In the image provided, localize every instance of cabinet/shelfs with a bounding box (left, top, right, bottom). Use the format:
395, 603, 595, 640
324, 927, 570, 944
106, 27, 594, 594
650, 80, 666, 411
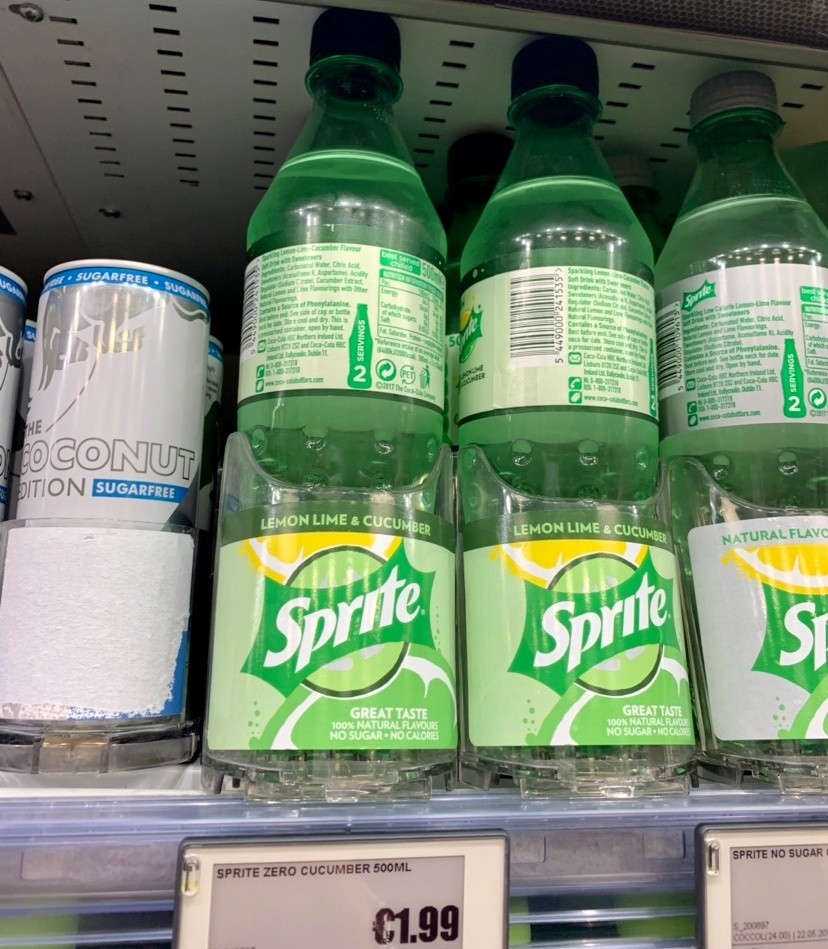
0, 0, 828, 949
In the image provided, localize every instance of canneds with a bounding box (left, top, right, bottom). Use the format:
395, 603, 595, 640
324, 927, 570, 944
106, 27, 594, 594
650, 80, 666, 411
0, 261, 225, 734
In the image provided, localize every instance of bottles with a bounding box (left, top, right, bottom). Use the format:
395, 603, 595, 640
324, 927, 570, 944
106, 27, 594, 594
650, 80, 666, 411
622, 185, 670, 265
237, 7, 450, 779
658, 68, 828, 753
447, 128, 514, 521
460, 34, 698, 782
